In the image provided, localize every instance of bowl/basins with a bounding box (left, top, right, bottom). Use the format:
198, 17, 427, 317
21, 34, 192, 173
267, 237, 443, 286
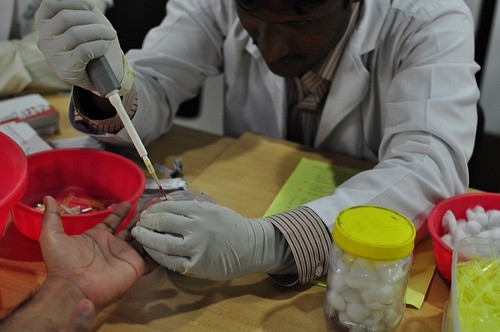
427, 193, 500, 281
13, 147, 146, 242
0, 131, 29, 238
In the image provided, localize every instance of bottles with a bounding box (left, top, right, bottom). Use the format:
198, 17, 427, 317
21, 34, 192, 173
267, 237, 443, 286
324, 206, 414, 332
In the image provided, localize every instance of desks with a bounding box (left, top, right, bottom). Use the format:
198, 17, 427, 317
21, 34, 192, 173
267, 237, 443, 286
1, 93, 480, 332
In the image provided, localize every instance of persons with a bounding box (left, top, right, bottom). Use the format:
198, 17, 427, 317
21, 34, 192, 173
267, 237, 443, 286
0, 196, 161, 332
34, 0, 480, 287
0, 0, 114, 96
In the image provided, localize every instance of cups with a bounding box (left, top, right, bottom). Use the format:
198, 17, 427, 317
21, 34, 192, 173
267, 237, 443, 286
450, 238, 500, 332
